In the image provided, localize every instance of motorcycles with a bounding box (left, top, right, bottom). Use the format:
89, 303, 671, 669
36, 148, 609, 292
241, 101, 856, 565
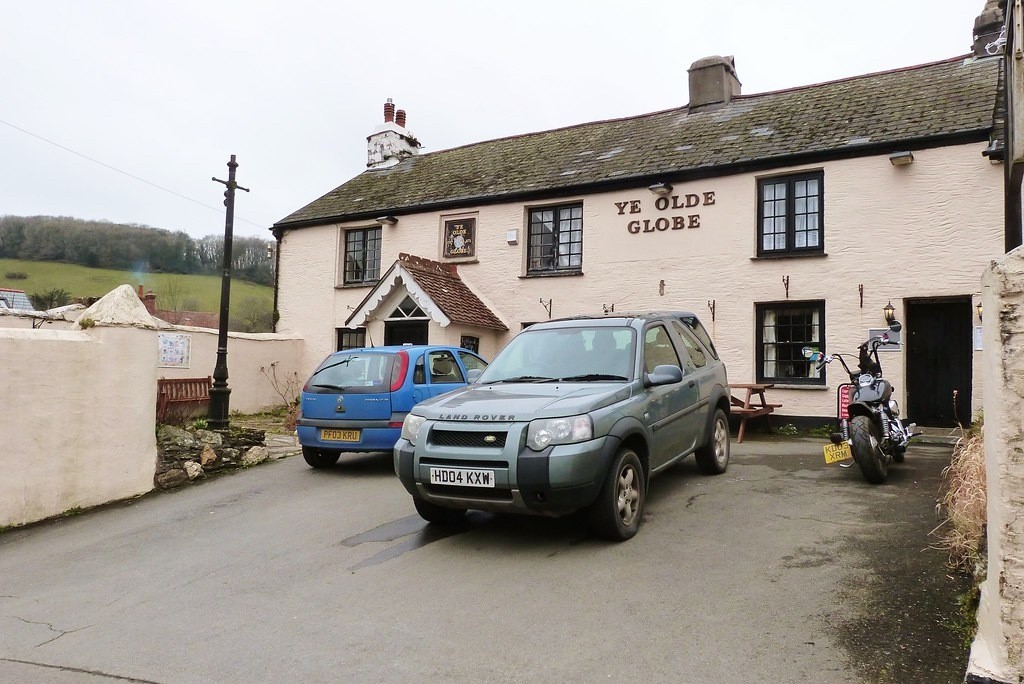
802, 321, 922, 484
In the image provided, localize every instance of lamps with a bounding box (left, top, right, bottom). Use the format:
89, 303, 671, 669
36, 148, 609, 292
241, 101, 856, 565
975, 302, 982, 322
889, 150, 914, 165
882, 301, 895, 326
649, 181, 674, 195
375, 216, 398, 225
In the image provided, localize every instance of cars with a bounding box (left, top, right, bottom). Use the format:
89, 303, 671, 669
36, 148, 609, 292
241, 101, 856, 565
297, 343, 488, 469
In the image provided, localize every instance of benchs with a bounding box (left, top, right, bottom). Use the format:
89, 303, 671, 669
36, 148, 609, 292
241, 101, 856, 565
729, 383, 774, 443
588, 328, 690, 375
157, 375, 212, 422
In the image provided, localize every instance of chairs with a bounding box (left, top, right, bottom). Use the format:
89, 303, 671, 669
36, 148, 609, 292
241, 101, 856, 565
432, 359, 457, 382
620, 341, 654, 377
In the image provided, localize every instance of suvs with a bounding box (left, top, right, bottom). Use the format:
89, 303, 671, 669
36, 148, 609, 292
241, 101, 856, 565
393, 310, 731, 542
